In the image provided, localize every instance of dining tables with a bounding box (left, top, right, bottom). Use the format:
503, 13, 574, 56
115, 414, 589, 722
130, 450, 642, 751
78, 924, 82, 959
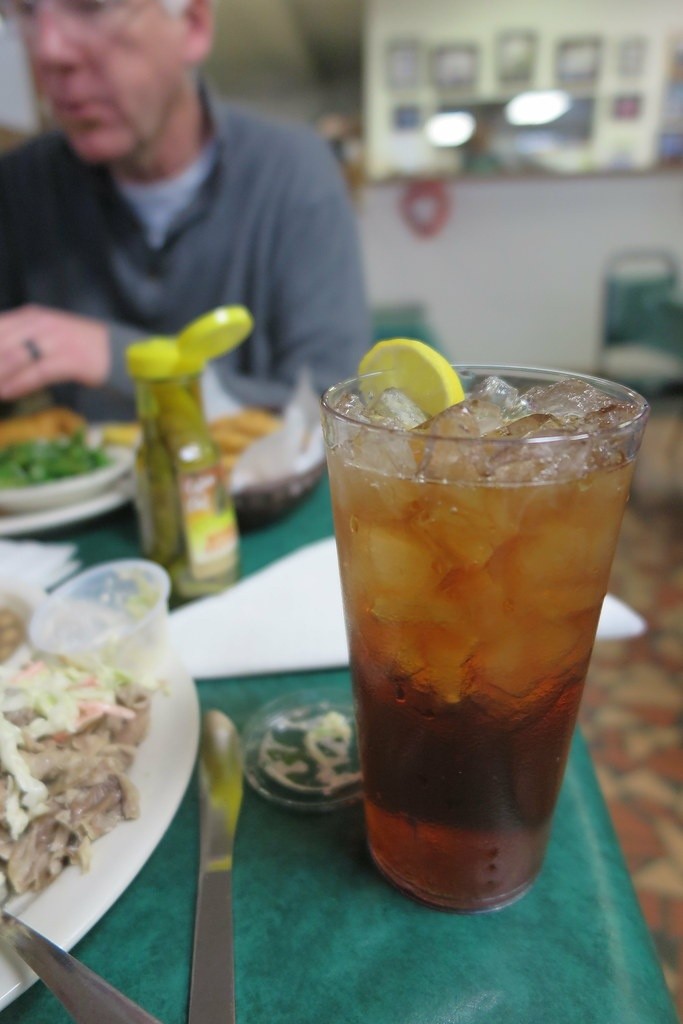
0, 316, 681, 1024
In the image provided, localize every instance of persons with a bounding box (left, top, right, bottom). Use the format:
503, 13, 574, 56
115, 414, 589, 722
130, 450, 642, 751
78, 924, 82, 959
0, 1, 372, 429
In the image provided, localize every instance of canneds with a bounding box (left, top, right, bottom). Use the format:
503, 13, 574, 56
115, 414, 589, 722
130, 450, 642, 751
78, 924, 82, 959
123, 309, 261, 597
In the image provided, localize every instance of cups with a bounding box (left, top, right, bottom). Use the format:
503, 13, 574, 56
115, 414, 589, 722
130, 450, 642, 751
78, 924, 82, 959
319, 363, 654, 912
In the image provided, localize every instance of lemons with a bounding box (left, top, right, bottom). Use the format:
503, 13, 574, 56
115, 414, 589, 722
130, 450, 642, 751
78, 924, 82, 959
358, 335, 463, 412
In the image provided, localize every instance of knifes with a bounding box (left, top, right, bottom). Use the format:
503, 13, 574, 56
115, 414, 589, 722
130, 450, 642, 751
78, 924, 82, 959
185, 706, 247, 1023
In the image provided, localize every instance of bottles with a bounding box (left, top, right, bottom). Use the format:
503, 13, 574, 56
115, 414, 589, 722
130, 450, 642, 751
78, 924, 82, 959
124, 333, 240, 602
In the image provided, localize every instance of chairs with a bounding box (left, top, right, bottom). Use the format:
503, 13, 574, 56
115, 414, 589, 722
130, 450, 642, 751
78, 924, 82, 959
588, 245, 681, 498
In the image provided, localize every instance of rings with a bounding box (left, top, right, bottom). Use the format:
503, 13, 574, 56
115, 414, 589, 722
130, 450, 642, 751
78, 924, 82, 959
24, 336, 43, 363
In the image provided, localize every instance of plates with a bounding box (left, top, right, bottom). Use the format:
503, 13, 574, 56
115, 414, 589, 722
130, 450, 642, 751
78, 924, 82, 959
0, 445, 133, 515
0, 472, 136, 543
0, 579, 202, 1014
